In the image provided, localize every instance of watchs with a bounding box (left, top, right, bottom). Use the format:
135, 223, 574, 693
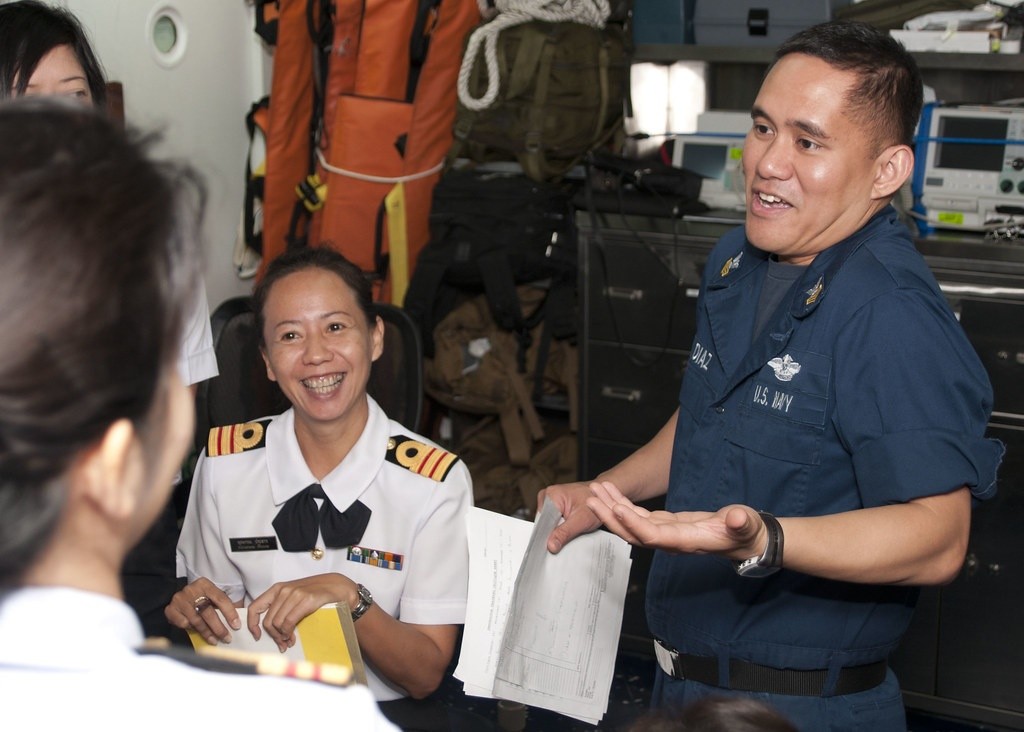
351, 584, 373, 622
730, 510, 784, 578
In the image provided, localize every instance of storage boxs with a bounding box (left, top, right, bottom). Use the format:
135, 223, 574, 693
692, 1, 854, 48
632, 0, 697, 44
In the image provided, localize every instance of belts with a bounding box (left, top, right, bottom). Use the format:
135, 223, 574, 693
650, 639, 893, 700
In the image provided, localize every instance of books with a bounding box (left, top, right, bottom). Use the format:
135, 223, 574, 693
185, 601, 370, 685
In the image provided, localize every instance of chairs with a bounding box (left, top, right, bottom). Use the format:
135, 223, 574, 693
119, 294, 423, 653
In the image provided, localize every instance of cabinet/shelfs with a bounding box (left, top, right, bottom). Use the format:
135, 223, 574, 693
885, 230, 1023, 728
576, 207, 747, 730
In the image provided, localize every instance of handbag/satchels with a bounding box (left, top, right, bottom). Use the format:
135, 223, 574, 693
454, 15, 633, 169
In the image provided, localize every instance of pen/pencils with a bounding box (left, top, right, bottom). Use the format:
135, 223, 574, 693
194, 585, 235, 614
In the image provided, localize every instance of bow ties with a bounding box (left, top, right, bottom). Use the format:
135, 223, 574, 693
272, 484, 372, 552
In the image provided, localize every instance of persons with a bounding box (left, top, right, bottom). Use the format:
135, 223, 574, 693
537, 19, 1006, 732
165, 245, 487, 731
1, 0, 220, 649
0, 94, 406, 730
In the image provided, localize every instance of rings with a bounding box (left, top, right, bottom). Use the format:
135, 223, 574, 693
192, 596, 209, 607
195, 600, 212, 614
